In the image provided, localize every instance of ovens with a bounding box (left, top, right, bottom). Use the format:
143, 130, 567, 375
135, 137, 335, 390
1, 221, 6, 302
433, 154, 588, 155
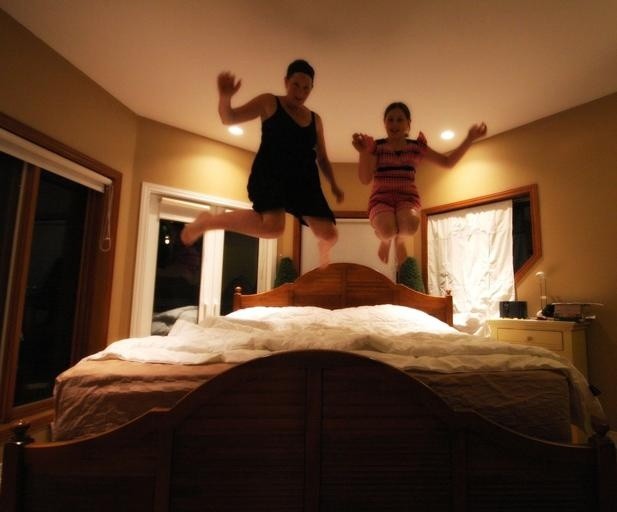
0, 262, 616, 512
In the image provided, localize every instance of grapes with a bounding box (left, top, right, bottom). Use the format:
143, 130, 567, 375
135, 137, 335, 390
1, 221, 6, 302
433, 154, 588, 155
482, 317, 589, 385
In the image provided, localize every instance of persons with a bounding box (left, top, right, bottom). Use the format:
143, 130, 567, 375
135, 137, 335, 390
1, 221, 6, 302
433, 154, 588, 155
180, 57, 344, 269
349, 100, 488, 270
